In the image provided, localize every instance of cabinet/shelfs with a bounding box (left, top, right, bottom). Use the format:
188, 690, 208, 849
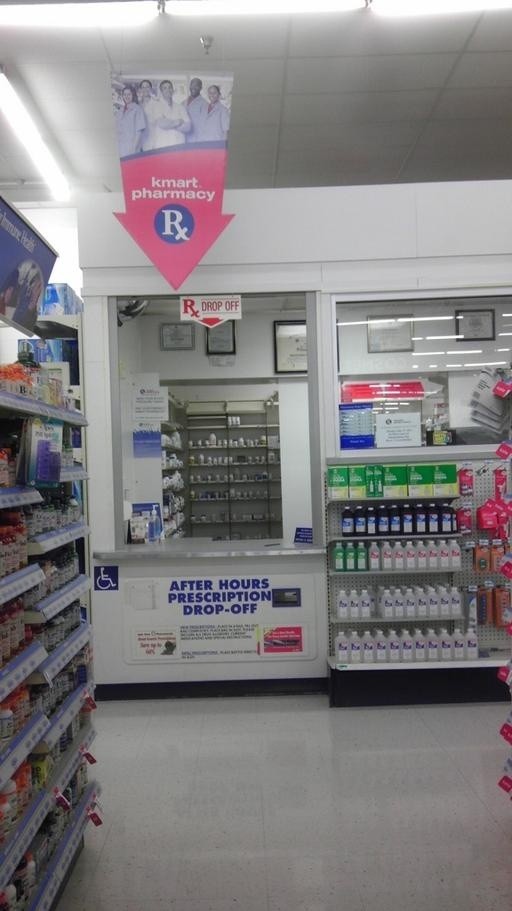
0, 191, 102, 911
134, 394, 284, 539
325, 493, 512, 707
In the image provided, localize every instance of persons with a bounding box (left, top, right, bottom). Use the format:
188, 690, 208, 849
190, 85, 229, 143
142, 80, 194, 151
116, 85, 146, 158
0, 276, 43, 330
179, 78, 209, 145
137, 79, 154, 105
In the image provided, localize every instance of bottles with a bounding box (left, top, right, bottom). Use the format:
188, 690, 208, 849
332, 501, 482, 662
1, 350, 93, 909
161, 426, 185, 544
423, 401, 450, 446
186, 415, 282, 540
